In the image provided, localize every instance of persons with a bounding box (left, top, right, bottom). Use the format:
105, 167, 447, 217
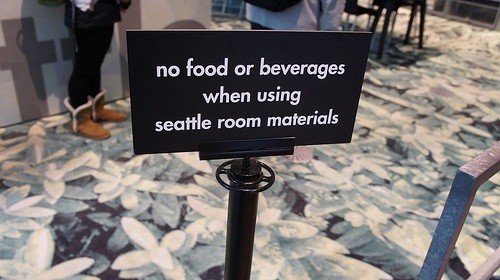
63, 0, 135, 141
245, 0, 347, 31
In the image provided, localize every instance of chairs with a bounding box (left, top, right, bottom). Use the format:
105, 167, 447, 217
342, 0, 428, 61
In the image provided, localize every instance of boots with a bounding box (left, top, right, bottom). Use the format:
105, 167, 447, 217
63, 95, 110, 141
87, 89, 129, 123
